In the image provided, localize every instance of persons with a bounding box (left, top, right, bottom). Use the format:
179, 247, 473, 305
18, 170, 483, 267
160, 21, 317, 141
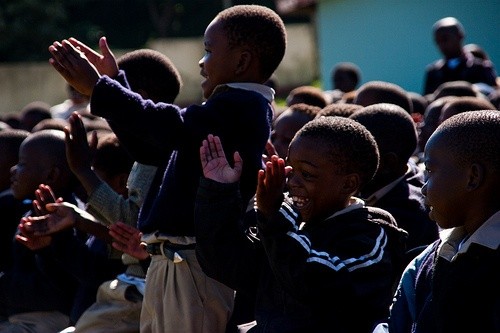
1, 5, 500, 333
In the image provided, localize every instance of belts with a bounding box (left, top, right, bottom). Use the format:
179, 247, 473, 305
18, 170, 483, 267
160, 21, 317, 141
144, 240, 196, 264
